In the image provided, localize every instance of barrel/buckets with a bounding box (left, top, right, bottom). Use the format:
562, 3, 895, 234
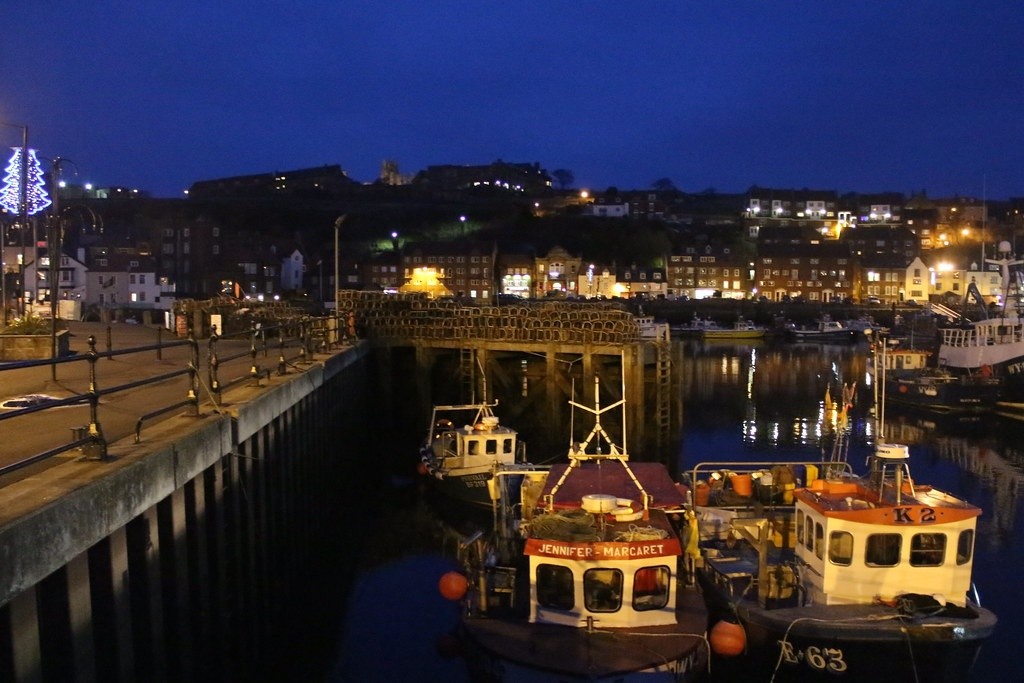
732, 475, 752, 496
696, 483, 710, 506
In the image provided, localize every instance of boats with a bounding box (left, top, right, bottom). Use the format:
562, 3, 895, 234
675, 339, 997, 683
634, 316, 667, 337
702, 266, 1023, 426
439, 350, 710, 683
670, 312, 727, 331
418, 357, 541, 522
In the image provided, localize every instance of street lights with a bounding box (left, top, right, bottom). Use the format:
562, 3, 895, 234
334, 214, 346, 313
